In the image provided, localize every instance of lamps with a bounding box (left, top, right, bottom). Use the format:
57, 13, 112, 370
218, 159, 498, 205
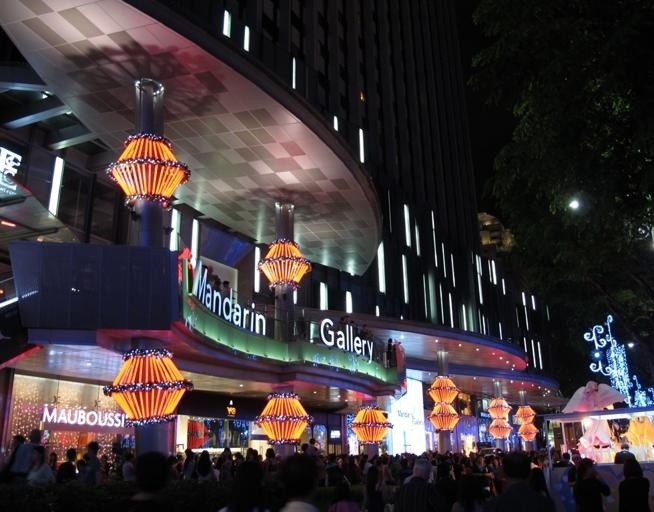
252, 392, 314, 445
104, 132, 313, 290
344, 405, 394, 444
101, 347, 193, 428
424, 376, 539, 442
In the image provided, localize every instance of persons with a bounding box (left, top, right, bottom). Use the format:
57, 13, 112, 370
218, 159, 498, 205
1, 428, 654, 512
199, 260, 232, 319
339, 314, 395, 368
